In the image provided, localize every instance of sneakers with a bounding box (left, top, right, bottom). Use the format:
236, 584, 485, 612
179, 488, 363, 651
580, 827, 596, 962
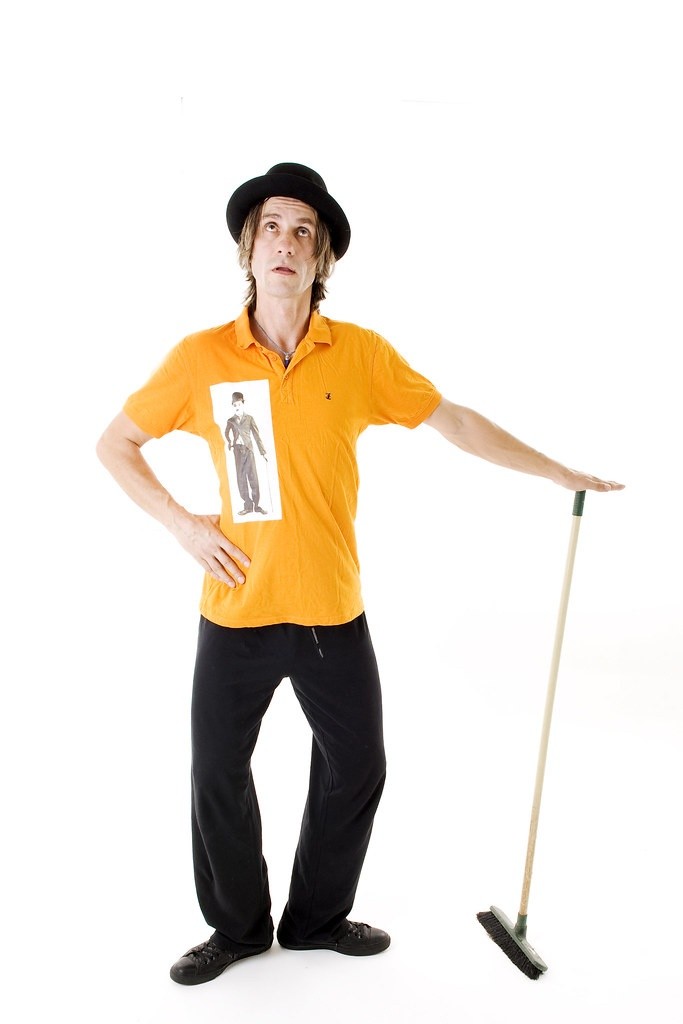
170, 937, 268, 985
279, 919, 391, 956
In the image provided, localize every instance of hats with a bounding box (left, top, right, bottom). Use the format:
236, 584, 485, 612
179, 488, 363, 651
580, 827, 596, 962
231, 392, 244, 404
225, 163, 351, 262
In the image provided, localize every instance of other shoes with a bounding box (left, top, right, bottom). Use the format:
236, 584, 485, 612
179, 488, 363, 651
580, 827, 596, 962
237, 508, 253, 514
255, 507, 268, 514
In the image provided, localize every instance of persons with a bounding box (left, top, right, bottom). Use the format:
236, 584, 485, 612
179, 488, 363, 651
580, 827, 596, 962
96, 163, 624, 987
224, 392, 270, 516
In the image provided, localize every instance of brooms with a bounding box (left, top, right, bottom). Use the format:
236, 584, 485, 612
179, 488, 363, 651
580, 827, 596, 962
474, 489, 585, 983
